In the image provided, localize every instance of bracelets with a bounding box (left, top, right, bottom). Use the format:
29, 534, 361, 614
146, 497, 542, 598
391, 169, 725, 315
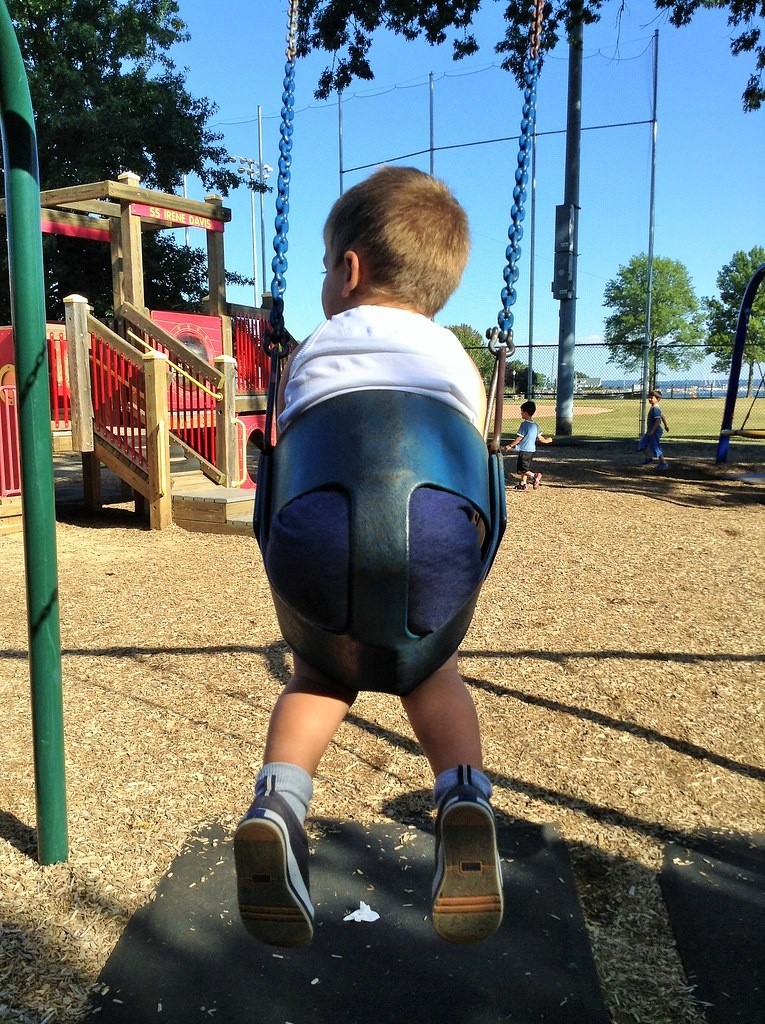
509, 444, 512, 447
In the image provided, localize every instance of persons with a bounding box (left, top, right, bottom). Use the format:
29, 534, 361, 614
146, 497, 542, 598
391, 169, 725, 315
638, 390, 671, 471
234, 168, 504, 949
504, 401, 553, 490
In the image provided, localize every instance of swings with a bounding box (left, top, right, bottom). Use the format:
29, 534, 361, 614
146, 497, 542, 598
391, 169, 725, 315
252, 1, 547, 682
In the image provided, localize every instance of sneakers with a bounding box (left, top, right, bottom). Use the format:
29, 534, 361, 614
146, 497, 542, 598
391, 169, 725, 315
654, 462, 669, 471
533, 472, 542, 490
639, 457, 653, 466
428, 763, 504, 947
513, 482, 528, 490
233, 774, 316, 949
